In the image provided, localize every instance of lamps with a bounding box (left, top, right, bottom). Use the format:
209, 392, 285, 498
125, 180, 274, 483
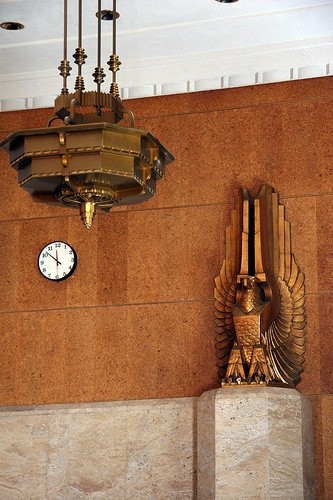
0, 0, 176, 231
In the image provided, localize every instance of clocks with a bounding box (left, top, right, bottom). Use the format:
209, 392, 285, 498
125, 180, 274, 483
37, 240, 78, 281
